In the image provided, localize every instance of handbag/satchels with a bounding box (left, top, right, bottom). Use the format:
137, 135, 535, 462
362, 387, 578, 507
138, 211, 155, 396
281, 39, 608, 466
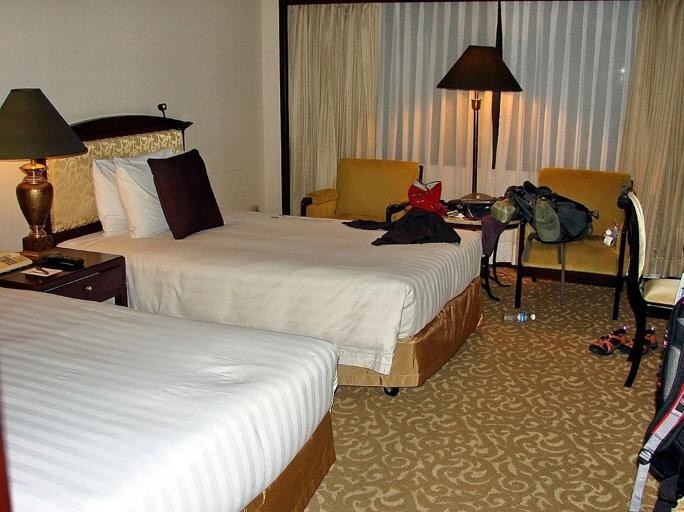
481, 199, 518, 257
408, 180, 446, 218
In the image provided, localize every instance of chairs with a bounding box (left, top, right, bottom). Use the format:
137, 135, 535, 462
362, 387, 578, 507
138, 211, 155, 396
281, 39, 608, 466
298, 157, 425, 223
616, 187, 683, 389
514, 167, 634, 321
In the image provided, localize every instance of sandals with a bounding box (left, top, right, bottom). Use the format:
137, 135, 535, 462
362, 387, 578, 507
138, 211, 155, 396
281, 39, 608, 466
590, 328, 628, 354
621, 330, 658, 354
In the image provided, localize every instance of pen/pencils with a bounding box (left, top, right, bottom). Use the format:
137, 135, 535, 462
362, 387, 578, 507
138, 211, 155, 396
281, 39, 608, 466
35, 265, 49, 274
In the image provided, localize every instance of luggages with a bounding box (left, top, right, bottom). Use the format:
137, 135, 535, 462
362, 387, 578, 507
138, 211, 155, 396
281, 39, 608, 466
659, 297, 684, 412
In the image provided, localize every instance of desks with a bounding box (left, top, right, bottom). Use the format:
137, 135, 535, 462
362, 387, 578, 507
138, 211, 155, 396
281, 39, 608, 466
0, 245, 128, 307
437, 211, 520, 301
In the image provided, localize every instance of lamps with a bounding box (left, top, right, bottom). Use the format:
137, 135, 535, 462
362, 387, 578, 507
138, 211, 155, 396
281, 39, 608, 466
433, 46, 525, 203
0, 89, 89, 259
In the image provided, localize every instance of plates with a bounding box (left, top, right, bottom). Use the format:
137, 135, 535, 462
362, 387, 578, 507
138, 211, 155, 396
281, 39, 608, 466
19, 266, 61, 277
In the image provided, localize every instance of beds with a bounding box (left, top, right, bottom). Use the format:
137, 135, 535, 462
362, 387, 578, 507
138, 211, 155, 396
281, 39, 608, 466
0, 288, 339, 511
30, 115, 483, 398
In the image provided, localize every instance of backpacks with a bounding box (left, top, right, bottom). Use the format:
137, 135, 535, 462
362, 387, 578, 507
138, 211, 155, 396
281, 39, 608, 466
638, 356, 684, 501
506, 181, 592, 244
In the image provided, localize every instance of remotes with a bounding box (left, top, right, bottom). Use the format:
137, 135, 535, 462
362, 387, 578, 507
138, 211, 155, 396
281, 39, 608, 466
42, 253, 84, 266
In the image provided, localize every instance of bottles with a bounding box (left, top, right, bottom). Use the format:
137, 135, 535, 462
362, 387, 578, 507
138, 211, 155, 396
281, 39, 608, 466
501, 313, 535, 323
605, 228, 615, 237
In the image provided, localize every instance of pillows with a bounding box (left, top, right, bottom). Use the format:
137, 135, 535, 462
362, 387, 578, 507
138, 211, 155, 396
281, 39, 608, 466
112, 148, 176, 242
90, 158, 132, 237
146, 147, 223, 240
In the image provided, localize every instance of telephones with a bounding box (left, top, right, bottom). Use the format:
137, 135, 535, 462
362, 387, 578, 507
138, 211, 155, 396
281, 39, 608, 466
1, 251, 33, 277
